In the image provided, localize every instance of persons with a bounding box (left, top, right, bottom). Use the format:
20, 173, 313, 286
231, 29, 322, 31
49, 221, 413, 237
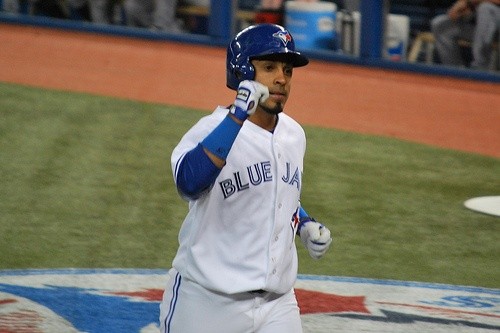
0, 0, 500, 74
158, 23, 331, 333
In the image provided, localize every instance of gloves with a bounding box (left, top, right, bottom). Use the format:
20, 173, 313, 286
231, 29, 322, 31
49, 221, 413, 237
296, 216, 332, 259
229, 79, 269, 121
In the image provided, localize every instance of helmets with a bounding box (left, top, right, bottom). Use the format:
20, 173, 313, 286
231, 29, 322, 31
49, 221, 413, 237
226, 22, 309, 91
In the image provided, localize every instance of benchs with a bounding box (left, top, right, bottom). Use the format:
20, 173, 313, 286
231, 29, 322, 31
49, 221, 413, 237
177, 5, 254, 30
408, 32, 500, 73
